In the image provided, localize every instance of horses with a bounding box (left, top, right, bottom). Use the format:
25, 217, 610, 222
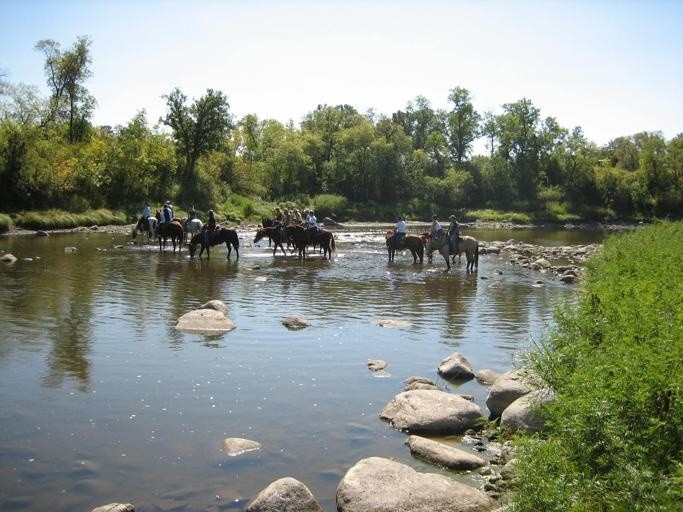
131, 209, 203, 254
422, 228, 478, 274
253, 216, 336, 261
386, 235, 424, 265
188, 228, 243, 263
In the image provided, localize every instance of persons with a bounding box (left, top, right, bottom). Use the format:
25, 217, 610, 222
431, 214, 442, 237
449, 215, 460, 254
392, 216, 407, 250
273, 206, 317, 245
204, 210, 216, 247
143, 200, 198, 236
